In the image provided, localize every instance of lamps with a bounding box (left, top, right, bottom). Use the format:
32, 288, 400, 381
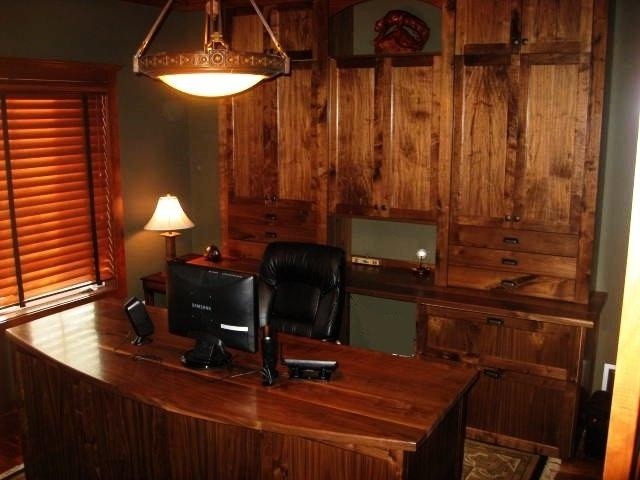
132, 0, 290, 98
143, 193, 195, 257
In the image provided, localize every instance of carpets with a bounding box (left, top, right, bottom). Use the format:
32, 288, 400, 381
462, 438, 562, 480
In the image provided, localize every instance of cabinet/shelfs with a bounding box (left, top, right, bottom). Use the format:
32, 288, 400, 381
203, 0, 353, 61
327, 51, 446, 220
441, 51, 606, 305
441, 0, 608, 55
217, 61, 332, 260
415, 291, 608, 462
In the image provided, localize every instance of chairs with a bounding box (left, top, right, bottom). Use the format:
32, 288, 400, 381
259, 241, 346, 342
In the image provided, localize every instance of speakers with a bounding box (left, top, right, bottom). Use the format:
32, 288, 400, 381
123, 297, 153, 339
257, 325, 278, 383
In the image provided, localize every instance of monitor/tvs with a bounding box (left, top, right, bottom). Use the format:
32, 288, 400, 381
165, 258, 259, 373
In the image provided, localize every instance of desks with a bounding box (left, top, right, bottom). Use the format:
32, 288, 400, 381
140, 272, 168, 306
5, 296, 479, 479
